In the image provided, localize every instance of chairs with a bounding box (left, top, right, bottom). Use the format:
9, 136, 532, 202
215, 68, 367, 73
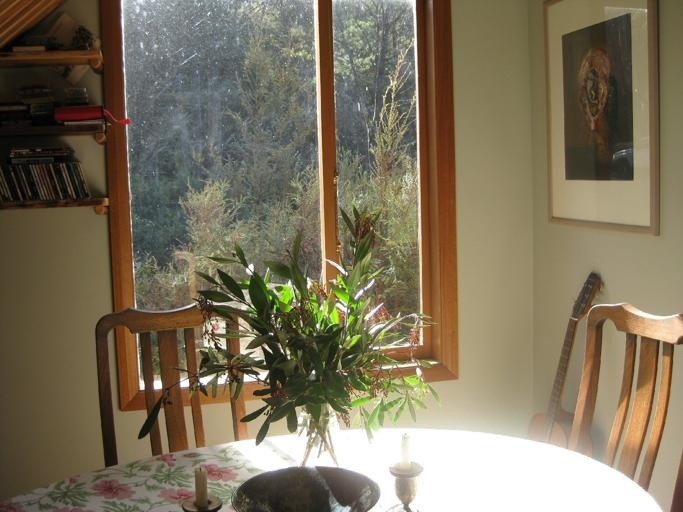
96, 299, 250, 467
566, 303, 683, 512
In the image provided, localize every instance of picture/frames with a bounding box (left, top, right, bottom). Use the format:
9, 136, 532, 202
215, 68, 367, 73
541, 1, 660, 238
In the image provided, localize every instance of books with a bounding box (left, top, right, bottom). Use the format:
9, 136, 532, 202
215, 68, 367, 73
0, 146, 90, 202
12, 11, 96, 51
0, 86, 112, 126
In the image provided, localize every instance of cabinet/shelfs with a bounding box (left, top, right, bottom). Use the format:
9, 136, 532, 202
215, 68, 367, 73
0, 51, 109, 216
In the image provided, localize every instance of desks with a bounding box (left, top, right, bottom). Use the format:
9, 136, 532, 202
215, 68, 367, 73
4, 426, 679, 512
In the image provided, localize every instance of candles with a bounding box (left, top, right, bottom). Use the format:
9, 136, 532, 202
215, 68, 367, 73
399, 433, 412, 472
195, 467, 208, 508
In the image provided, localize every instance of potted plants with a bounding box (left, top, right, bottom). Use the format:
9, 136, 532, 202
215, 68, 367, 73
134, 205, 443, 466
